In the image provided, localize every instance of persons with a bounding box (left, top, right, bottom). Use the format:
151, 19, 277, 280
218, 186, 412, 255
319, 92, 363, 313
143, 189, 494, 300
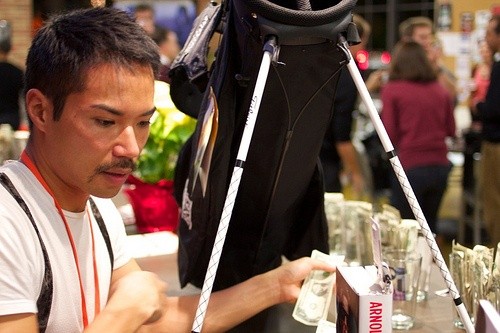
380, 40, 457, 236
0, 0, 181, 131
0, 6, 336, 333
399, 13, 500, 248
320, 13, 371, 196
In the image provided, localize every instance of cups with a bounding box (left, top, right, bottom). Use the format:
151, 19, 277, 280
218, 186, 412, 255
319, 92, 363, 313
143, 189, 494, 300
326, 197, 435, 301
449, 252, 500, 328
381, 251, 422, 330
